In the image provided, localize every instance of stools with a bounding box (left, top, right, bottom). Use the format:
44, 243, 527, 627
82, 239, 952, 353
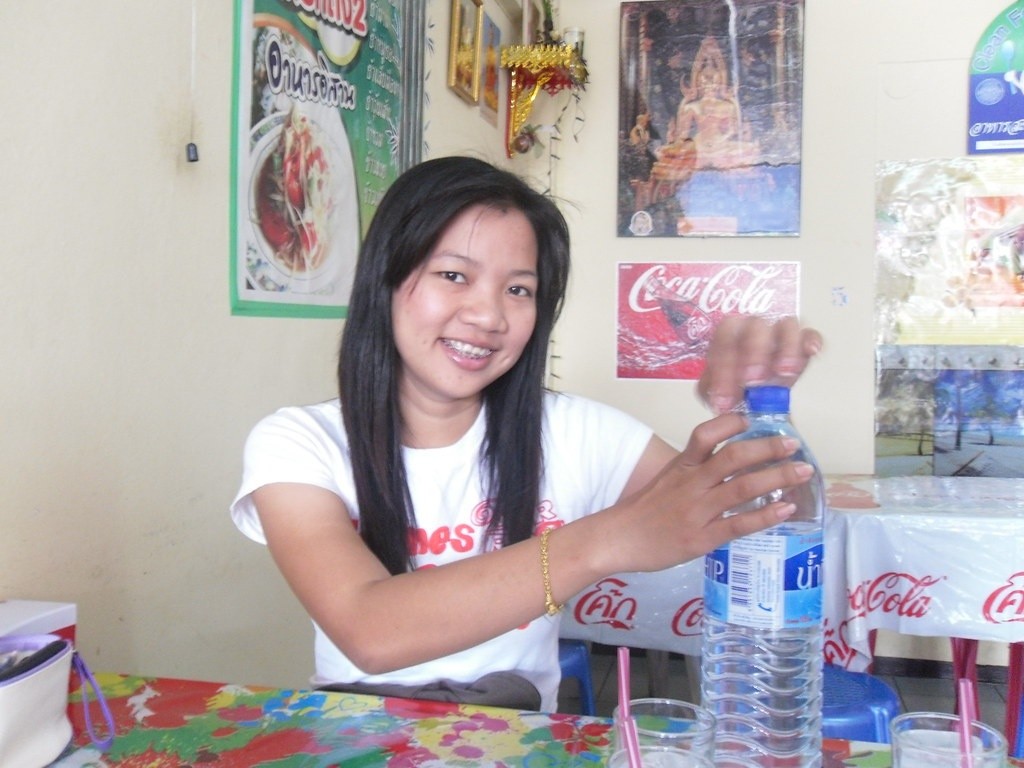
559, 636, 594, 717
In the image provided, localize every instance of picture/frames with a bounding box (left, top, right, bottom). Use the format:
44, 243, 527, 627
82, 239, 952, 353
481, 11, 501, 121
448, 1, 485, 106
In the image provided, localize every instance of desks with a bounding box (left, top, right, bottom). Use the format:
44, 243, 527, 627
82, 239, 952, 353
551, 476, 1024, 755
44, 670, 1021, 766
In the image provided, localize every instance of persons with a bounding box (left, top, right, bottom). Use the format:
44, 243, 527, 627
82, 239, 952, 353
227, 157, 824, 716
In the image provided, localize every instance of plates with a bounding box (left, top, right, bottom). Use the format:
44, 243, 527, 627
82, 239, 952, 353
247, 111, 342, 294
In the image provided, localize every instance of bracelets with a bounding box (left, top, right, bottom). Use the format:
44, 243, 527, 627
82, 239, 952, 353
538, 530, 570, 615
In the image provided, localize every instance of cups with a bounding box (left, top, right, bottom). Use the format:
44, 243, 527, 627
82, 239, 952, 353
604, 697, 719, 768
889, 710, 1008, 765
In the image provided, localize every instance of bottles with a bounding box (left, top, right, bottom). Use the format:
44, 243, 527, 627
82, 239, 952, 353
698, 382, 825, 768
